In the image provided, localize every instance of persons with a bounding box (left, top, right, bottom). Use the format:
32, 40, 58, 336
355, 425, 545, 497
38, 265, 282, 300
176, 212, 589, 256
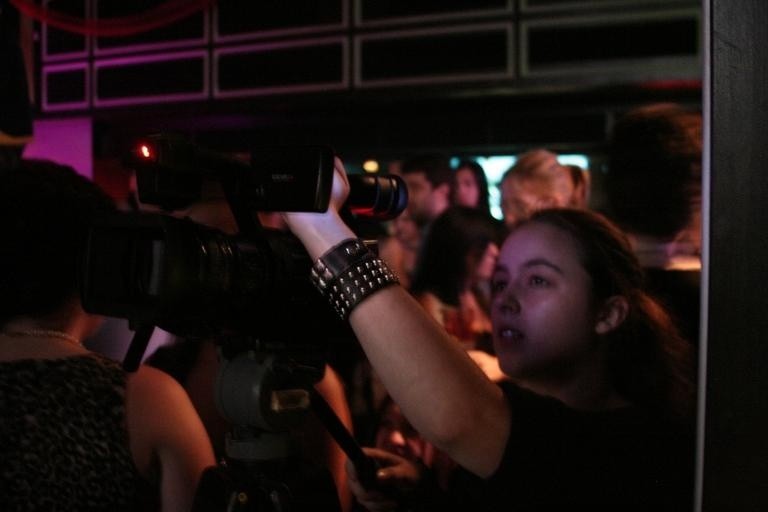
0, 98, 706, 512
